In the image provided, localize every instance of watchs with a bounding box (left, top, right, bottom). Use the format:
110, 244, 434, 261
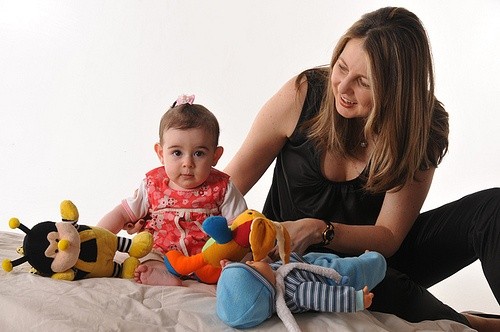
321, 220, 335, 246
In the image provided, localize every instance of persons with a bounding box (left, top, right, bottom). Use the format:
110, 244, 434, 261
96, 94, 248, 287
122, 6, 500, 332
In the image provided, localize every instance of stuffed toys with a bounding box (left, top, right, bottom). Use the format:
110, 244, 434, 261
164, 209, 292, 285
216, 249, 386, 332
3, 200, 153, 281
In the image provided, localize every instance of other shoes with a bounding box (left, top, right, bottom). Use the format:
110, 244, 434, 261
460, 311, 500, 332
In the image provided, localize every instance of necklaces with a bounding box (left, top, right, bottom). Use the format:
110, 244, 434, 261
359, 127, 370, 149
